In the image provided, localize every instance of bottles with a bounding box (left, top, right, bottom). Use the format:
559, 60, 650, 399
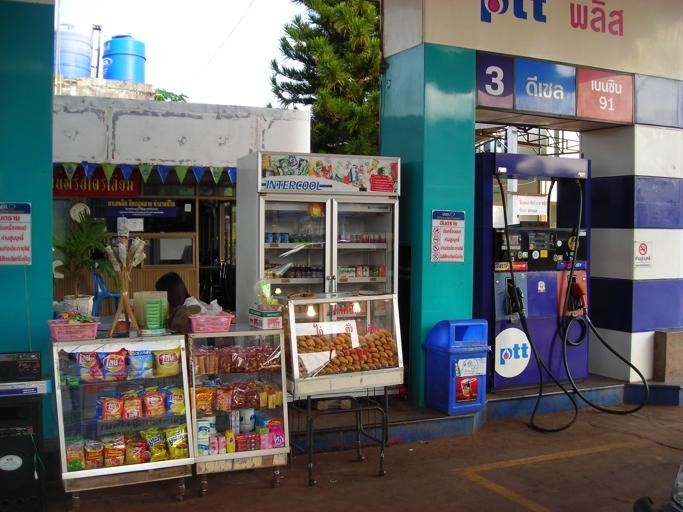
265, 231, 386, 277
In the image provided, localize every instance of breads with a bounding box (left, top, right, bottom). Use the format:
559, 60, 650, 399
284, 329, 398, 379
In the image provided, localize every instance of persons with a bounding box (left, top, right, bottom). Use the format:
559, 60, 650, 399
154, 272, 208, 315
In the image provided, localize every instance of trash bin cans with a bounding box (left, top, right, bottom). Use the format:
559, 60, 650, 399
421, 318, 492, 417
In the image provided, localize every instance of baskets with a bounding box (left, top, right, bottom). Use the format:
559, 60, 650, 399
186, 307, 236, 334
46, 316, 102, 343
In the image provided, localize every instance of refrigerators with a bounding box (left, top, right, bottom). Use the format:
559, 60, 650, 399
237, 150, 402, 403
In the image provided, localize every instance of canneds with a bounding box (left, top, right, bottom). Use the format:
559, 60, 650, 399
349, 164, 358, 182
279, 159, 293, 175
299, 159, 307, 176
264, 232, 289, 243
